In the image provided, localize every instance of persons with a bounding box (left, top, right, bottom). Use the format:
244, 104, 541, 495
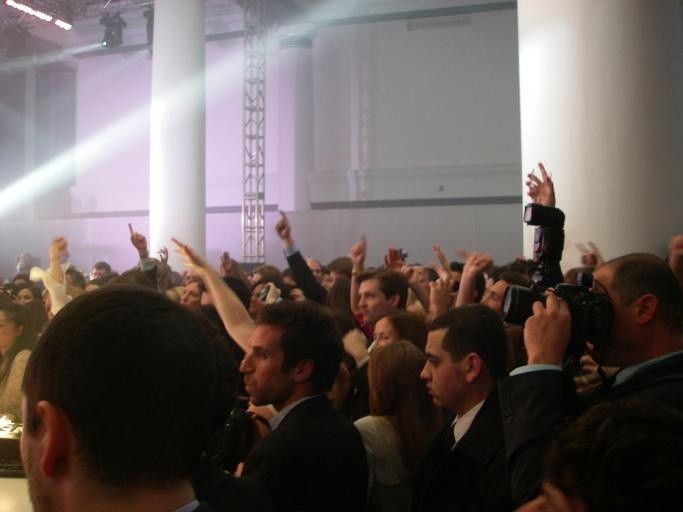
0, 162, 682, 512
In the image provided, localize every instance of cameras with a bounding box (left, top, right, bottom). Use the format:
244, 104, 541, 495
501, 203, 614, 343
259, 287, 268, 300
